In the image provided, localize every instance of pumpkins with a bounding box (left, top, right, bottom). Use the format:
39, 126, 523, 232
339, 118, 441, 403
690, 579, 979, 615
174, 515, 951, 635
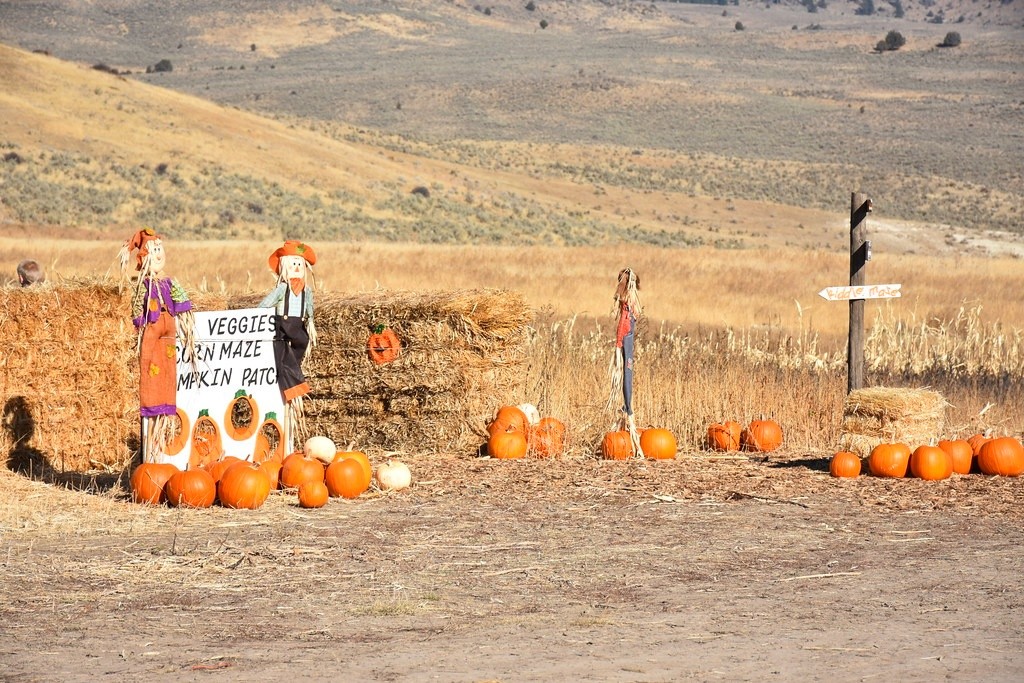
707, 418, 742, 451
829, 448, 861, 478
485, 403, 566, 460
869, 429, 1024, 480
601, 424, 677, 459
128, 436, 411, 510
742, 413, 782, 453
